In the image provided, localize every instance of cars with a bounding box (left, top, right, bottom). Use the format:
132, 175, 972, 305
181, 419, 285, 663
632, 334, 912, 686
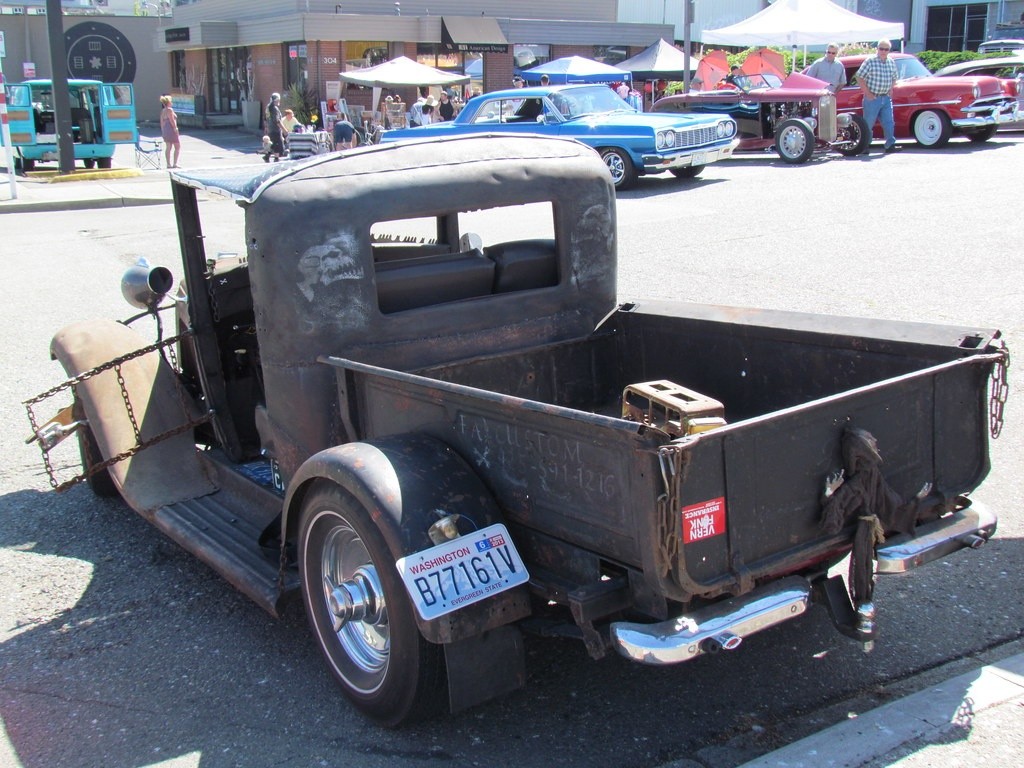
932, 57, 1024, 130
378, 84, 743, 191
798, 52, 1020, 150
648, 71, 872, 164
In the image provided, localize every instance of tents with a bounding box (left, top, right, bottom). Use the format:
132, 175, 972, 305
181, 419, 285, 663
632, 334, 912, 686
700, 0, 904, 72
337, 55, 470, 119
449, 58, 483, 80
615, 37, 700, 104
522, 56, 633, 92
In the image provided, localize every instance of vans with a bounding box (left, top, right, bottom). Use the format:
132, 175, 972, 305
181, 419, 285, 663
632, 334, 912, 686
3, 78, 139, 172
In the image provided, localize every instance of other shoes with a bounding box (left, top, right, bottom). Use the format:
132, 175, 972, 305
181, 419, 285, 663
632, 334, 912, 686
885, 145, 903, 153
274, 159, 279, 162
262, 155, 270, 163
863, 148, 869, 154
168, 165, 172, 168
173, 166, 181, 168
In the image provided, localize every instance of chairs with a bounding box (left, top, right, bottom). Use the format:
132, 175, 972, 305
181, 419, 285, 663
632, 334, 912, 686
356, 124, 382, 146
72, 108, 92, 141
248, 239, 559, 458
135, 130, 163, 170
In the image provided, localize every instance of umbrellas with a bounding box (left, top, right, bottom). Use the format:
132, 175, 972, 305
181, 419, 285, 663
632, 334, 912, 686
695, 51, 729, 90
742, 48, 786, 86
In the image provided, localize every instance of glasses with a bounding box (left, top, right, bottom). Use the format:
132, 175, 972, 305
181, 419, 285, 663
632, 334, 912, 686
827, 51, 836, 55
879, 47, 889, 51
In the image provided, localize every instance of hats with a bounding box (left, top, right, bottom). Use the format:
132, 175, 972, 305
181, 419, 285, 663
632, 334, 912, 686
514, 77, 525, 82
691, 77, 703, 85
422, 96, 439, 107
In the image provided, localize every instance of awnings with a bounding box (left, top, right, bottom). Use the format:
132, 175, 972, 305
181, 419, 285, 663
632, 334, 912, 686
442, 15, 508, 54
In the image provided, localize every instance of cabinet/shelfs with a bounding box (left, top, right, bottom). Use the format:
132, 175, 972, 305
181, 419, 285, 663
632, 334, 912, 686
381, 102, 405, 128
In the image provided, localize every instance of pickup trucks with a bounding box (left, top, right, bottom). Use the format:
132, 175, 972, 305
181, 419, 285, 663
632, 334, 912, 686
20, 131, 1010, 730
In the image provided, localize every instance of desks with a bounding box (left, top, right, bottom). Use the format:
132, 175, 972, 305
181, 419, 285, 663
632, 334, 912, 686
288, 131, 327, 160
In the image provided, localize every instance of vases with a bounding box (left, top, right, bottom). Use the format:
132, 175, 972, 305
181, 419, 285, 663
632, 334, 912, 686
194, 95, 206, 115
241, 100, 261, 129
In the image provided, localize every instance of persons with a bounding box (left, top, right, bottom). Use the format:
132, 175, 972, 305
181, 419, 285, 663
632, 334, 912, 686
160, 96, 180, 167
648, 82, 665, 105
855, 40, 903, 153
507, 77, 526, 110
807, 45, 847, 96
335, 121, 356, 151
617, 82, 629, 99
256, 93, 300, 163
385, 86, 479, 128
541, 75, 549, 85
727, 65, 743, 87
690, 78, 704, 92
